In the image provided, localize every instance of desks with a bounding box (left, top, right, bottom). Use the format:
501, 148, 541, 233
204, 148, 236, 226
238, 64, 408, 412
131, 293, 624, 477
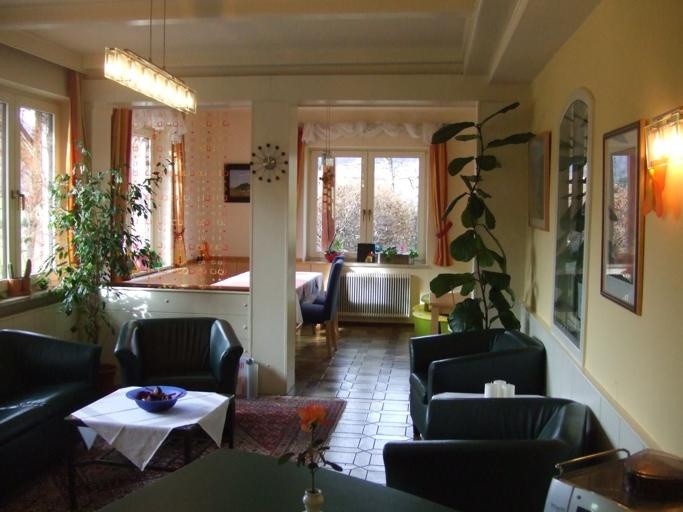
428, 292, 468, 335
212, 264, 321, 331
100, 448, 449, 510
63, 392, 234, 494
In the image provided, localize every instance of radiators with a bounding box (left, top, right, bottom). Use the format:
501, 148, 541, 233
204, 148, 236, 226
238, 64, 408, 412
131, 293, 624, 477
317, 273, 413, 319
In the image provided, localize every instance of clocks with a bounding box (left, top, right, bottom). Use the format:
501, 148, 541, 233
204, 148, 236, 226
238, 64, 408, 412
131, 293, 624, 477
249, 142, 288, 183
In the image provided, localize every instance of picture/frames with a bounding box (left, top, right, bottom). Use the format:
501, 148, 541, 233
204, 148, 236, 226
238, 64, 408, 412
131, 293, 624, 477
223, 163, 249, 204
522, 131, 552, 232
599, 116, 649, 318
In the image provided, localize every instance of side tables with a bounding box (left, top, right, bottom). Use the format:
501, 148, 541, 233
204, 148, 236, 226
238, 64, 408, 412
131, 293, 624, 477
546, 447, 683, 512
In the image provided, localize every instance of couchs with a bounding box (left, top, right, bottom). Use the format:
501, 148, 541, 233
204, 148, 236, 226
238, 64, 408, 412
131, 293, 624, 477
0, 328, 103, 463
405, 328, 547, 439
111, 315, 245, 447
378, 393, 597, 511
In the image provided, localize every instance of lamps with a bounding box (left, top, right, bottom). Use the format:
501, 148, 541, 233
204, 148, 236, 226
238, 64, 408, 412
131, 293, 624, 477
103, 0, 199, 116
640, 108, 683, 219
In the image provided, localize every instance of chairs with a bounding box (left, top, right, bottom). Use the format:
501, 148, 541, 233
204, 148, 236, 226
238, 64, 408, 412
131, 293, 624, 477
301, 260, 343, 359
312, 254, 343, 341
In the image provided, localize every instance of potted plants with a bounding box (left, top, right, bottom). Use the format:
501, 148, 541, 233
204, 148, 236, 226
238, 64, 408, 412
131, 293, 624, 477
35, 140, 175, 388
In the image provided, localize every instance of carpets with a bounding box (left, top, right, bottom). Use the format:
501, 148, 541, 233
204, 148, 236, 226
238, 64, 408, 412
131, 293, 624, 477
0, 395, 346, 511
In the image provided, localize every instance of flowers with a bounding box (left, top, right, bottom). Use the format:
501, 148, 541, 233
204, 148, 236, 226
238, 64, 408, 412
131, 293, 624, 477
275, 404, 343, 511
